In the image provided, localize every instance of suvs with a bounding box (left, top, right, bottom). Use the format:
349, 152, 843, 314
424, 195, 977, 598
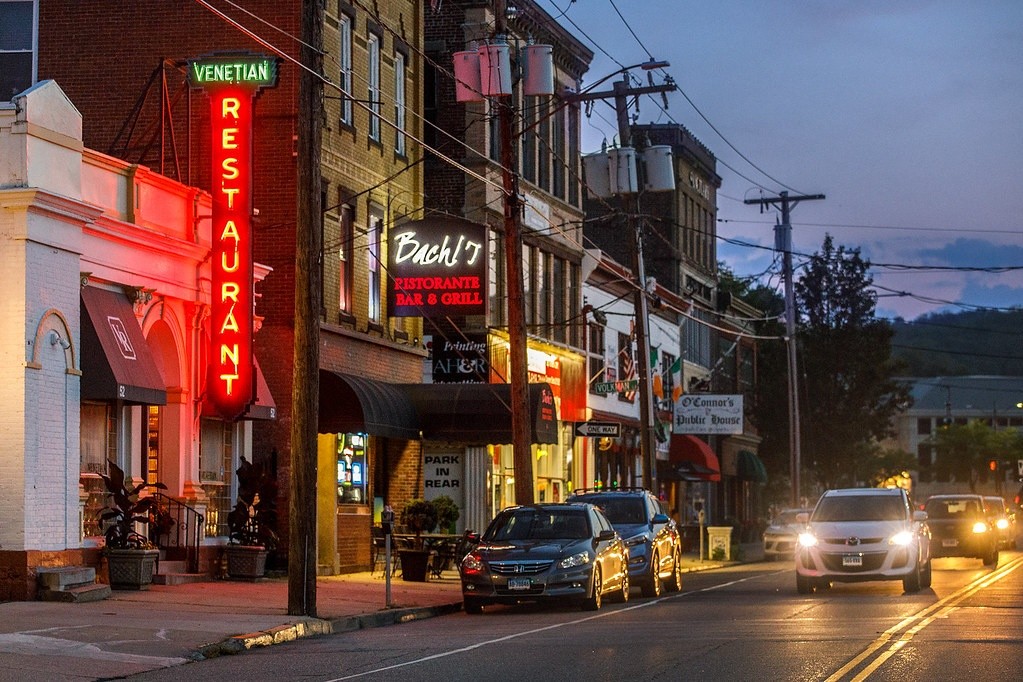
567, 486, 682, 598
792, 488, 932, 595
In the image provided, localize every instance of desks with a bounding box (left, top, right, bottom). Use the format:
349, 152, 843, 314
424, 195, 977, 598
392, 533, 463, 580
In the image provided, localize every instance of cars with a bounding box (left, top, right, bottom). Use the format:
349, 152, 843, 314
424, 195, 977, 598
763, 508, 812, 561
985, 497, 1018, 549
459, 507, 629, 610
919, 496, 1000, 565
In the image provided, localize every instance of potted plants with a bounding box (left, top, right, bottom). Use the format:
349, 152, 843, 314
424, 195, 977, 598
93, 458, 168, 591
398, 498, 439, 582
222, 456, 278, 579
431, 495, 459, 571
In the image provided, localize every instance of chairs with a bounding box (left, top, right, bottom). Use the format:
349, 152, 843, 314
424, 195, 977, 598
435, 528, 480, 579
370, 524, 404, 578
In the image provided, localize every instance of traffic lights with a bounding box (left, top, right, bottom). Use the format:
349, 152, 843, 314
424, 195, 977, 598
990, 459, 999, 472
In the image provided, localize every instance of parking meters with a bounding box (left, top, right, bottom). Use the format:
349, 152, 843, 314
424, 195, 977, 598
381, 507, 396, 607
696, 511, 706, 562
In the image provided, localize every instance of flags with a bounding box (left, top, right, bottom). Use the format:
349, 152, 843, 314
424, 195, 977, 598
623, 338, 638, 401
670, 357, 683, 402
651, 350, 664, 400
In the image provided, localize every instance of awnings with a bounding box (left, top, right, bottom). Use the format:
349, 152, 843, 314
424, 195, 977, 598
738, 451, 768, 482
80, 285, 167, 407
200, 353, 277, 421
318, 369, 559, 446
669, 432, 721, 482
